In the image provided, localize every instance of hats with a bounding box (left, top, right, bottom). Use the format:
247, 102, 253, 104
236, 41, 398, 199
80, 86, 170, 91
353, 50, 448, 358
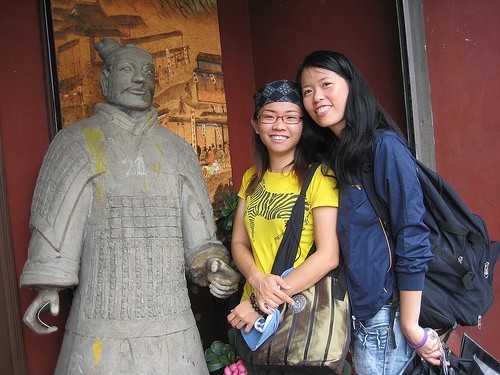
253, 80, 305, 113
240, 308, 280, 351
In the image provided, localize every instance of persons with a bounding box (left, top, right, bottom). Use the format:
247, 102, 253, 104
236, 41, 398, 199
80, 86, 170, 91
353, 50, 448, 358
228, 80, 340, 375
20, 38, 241, 375
296, 50, 450, 375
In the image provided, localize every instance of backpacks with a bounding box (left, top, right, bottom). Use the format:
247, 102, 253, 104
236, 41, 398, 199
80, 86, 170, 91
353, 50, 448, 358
361, 129, 500, 330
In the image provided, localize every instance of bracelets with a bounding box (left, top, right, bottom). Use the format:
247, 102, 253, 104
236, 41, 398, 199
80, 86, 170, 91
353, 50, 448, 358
407, 330, 428, 348
250, 293, 263, 314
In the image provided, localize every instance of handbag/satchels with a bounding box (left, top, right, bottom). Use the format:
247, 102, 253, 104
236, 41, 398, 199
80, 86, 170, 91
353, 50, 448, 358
235, 276, 351, 375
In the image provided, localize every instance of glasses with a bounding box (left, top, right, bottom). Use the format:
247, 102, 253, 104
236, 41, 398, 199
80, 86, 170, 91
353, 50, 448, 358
256, 115, 305, 124
